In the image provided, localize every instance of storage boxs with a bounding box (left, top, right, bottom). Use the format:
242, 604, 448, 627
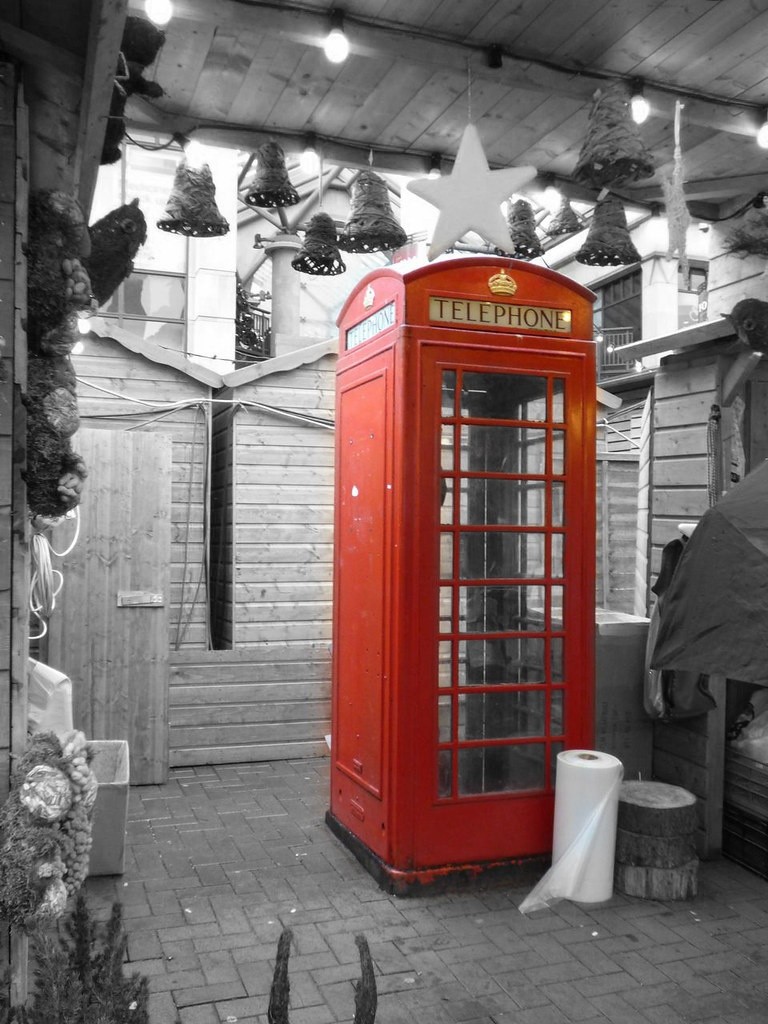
526, 607, 653, 781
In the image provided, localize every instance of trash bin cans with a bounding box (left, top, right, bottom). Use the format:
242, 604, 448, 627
89, 740, 129, 876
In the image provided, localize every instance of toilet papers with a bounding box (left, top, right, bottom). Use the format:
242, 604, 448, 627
518, 747, 623, 912
